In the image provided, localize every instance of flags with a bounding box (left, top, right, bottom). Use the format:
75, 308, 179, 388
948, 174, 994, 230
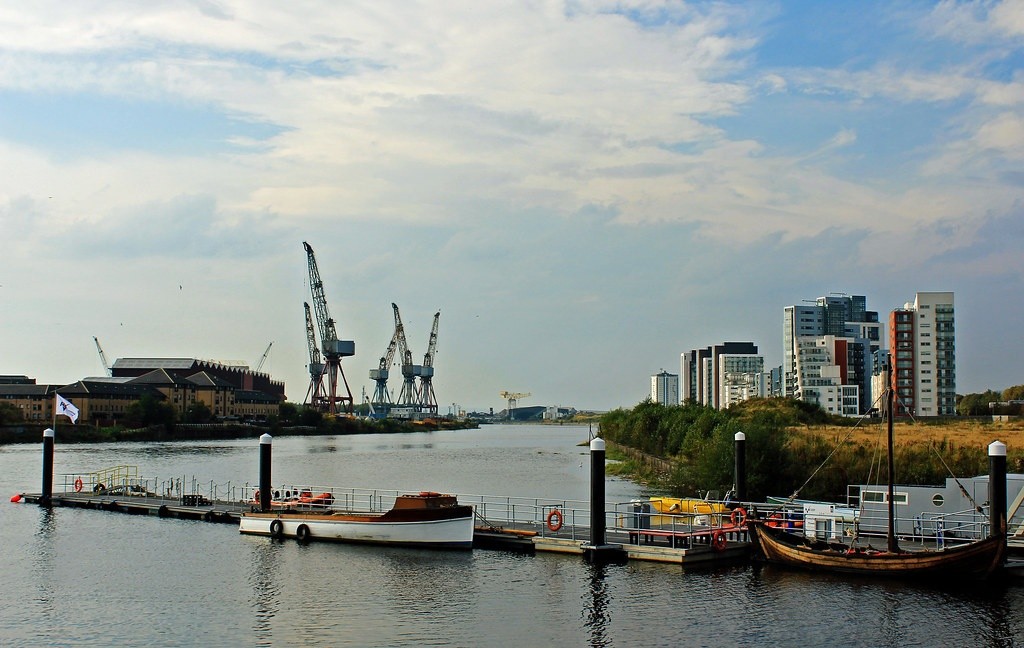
56, 393, 79, 425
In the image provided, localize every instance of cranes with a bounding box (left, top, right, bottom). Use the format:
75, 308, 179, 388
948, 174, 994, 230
94, 335, 112, 378
302, 240, 356, 419
370, 322, 404, 408
255, 341, 276, 372
303, 302, 327, 417
391, 303, 422, 417
415, 310, 445, 419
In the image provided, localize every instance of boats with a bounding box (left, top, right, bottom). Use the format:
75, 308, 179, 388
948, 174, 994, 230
237, 488, 477, 550
240, 488, 334, 510
640, 461, 1024, 570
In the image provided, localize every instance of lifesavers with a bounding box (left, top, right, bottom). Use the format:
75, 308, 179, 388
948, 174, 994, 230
75, 479, 82, 492
730, 508, 747, 527
546, 510, 563, 532
713, 529, 727, 552
255, 490, 261, 505
